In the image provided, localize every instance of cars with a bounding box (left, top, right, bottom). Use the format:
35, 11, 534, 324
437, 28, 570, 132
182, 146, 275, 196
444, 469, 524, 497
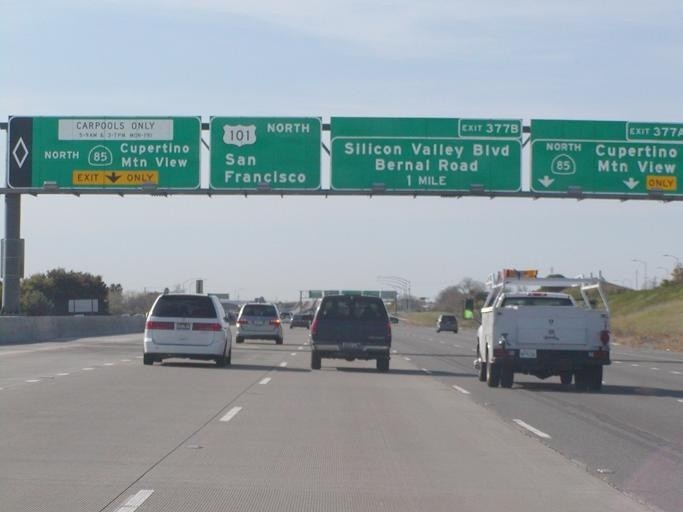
290, 314, 312, 329
279, 312, 293, 323
132, 313, 145, 317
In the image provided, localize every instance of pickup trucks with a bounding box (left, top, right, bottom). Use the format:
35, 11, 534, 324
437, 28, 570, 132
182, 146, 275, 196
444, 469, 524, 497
464, 268, 612, 388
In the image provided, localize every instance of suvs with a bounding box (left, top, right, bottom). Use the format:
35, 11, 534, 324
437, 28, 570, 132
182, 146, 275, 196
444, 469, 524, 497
308, 295, 398, 370
436, 315, 457, 333
235, 302, 283, 345
142, 287, 232, 365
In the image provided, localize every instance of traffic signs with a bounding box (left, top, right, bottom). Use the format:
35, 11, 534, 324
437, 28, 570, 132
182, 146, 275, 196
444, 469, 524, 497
32, 116, 200, 191
324, 290, 340, 296
308, 290, 322, 298
530, 119, 683, 195
209, 117, 323, 193
329, 116, 521, 193
341, 290, 362, 295
362, 291, 380, 297
380, 290, 397, 300
